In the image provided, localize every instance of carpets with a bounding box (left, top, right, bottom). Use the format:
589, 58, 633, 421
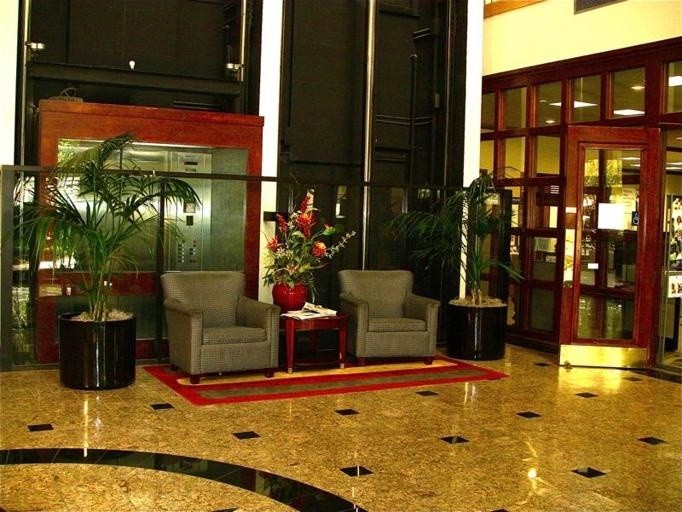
144, 353, 509, 406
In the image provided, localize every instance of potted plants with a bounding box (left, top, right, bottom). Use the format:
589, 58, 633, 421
16, 132, 197, 391
372, 165, 523, 360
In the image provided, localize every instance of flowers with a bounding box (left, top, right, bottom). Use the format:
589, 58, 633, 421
264, 193, 356, 300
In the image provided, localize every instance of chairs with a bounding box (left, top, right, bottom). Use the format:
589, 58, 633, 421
161, 271, 280, 384
339, 270, 440, 366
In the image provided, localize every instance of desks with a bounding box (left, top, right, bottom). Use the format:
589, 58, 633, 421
281, 312, 351, 374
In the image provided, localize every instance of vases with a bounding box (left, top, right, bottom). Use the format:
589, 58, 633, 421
271, 282, 310, 312
